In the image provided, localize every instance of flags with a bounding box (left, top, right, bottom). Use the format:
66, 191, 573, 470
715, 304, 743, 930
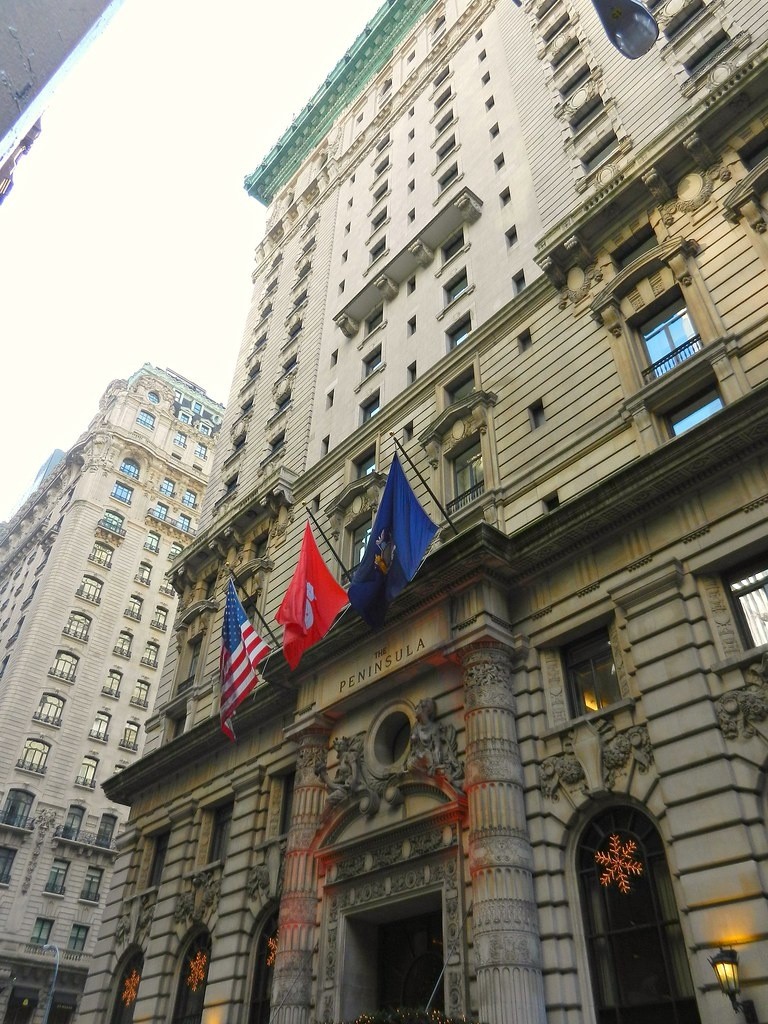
348, 453, 440, 631
219, 577, 273, 744
274, 518, 350, 674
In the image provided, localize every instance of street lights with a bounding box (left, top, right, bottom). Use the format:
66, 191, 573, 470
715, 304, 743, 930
43, 944, 60, 1024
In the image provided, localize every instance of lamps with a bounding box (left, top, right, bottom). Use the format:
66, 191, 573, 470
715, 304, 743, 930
706, 946, 760, 1024
